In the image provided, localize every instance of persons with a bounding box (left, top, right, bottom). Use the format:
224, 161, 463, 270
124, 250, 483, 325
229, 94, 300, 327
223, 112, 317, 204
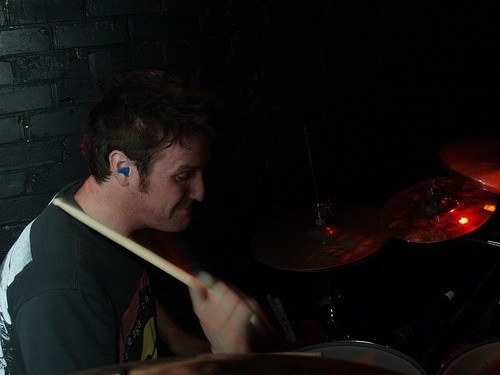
1, 68, 273, 375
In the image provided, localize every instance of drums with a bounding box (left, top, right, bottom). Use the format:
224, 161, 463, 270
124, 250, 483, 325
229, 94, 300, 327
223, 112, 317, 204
290, 337, 427, 375
434, 336, 500, 375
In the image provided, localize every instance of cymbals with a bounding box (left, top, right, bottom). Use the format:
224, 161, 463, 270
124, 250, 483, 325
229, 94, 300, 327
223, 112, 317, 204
253, 200, 397, 271
384, 171, 497, 246
440, 138, 500, 195
